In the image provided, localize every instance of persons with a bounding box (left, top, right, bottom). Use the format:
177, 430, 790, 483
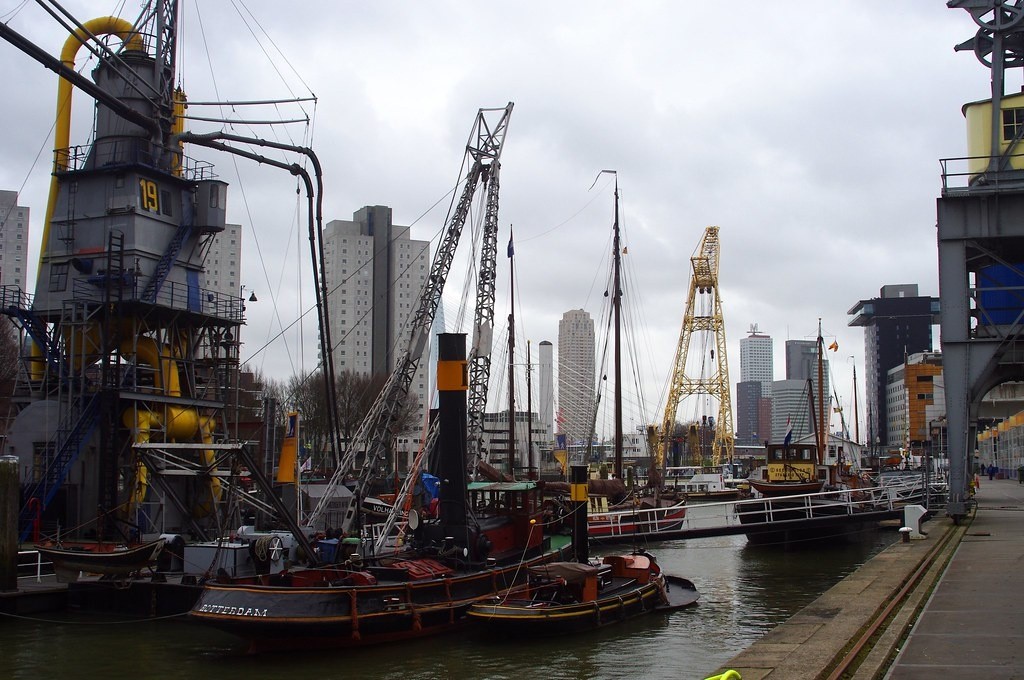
981, 464, 985, 475
987, 464, 994, 480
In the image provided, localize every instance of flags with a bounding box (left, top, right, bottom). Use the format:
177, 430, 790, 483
508, 236, 514, 258
834, 407, 843, 412
783, 419, 792, 451
828, 342, 838, 352
300, 457, 311, 473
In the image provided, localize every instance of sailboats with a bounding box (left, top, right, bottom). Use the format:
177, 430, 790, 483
0, 102, 837, 625
